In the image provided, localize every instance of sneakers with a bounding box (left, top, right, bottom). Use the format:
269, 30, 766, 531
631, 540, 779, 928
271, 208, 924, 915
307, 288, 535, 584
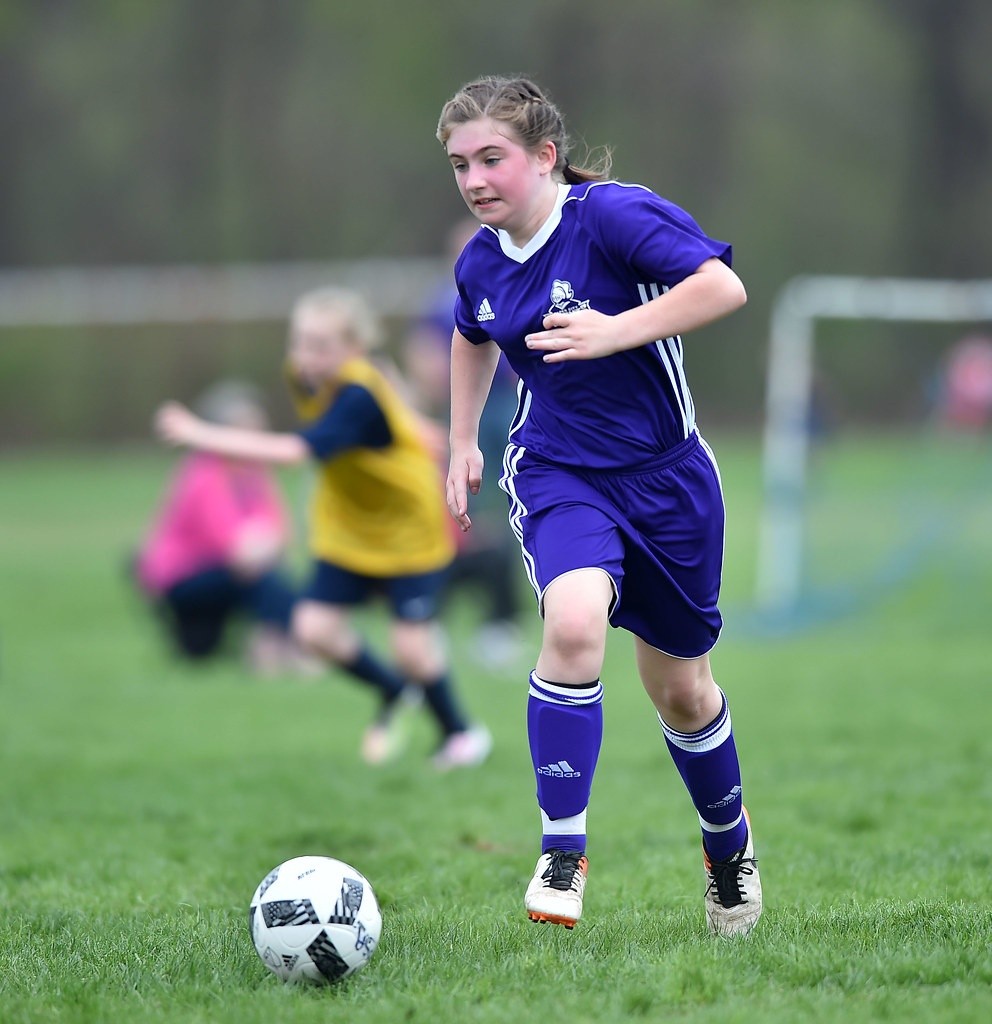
702, 804, 762, 940
524, 851, 590, 929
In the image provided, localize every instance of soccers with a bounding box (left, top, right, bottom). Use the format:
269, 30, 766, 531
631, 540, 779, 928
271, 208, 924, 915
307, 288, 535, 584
244, 852, 386, 990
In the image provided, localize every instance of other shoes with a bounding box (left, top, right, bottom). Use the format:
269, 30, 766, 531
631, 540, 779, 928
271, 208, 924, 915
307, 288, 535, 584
432, 725, 488, 768
364, 728, 396, 763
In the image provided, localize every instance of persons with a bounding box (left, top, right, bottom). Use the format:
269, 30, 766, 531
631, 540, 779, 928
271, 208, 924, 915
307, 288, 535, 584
135, 220, 520, 772
436, 75, 763, 939
